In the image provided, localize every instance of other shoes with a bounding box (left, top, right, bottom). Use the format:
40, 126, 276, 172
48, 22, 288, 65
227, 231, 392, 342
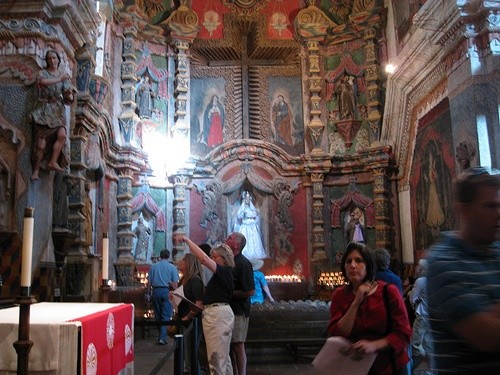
157, 340, 168, 345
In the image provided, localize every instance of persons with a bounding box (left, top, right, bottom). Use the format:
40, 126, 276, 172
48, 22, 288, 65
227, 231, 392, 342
346, 214, 363, 242
147, 249, 178, 345
174, 235, 235, 375
193, 183, 218, 229
225, 232, 255, 375
336, 75, 354, 120
325, 241, 413, 375
28, 50, 72, 183
133, 217, 151, 262
198, 244, 213, 286
411, 166, 500, 375
136, 73, 155, 119
168, 254, 211, 375
375, 249, 402, 294
249, 257, 274, 305
237, 194, 267, 258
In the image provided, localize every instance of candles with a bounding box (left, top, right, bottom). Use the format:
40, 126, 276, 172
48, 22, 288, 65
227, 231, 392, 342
137, 272, 183, 318
333, 276, 337, 280
325, 277, 328, 279
321, 272, 325, 276
102, 232, 109, 279
337, 280, 340, 284
323, 281, 326, 283
329, 276, 333, 280
327, 281, 331, 284
264, 275, 301, 283
320, 277, 324, 280
335, 272, 339, 276
332, 280, 335, 284
330, 272, 333, 276
21, 207, 34, 287
341, 280, 344, 284
326, 272, 329, 276
338, 272, 342, 275
338, 276, 341, 280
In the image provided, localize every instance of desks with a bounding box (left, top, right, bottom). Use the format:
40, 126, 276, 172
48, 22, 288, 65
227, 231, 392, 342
0, 302, 135, 375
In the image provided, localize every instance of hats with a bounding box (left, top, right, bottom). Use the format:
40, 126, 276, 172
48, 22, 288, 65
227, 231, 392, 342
374, 248, 390, 270
249, 258, 264, 271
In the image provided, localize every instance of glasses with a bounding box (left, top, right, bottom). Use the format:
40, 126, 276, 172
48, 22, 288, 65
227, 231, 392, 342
463, 165, 500, 176
217, 244, 225, 248
